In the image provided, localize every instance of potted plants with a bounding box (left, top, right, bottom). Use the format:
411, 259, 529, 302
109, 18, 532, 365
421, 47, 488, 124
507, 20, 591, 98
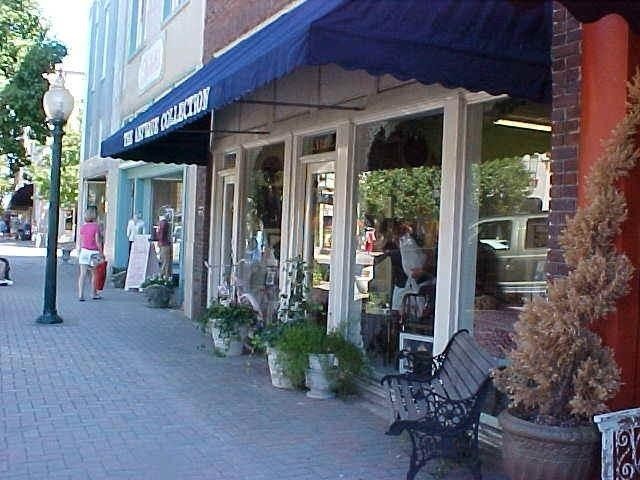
111, 265, 126, 288
487, 63, 640, 480
194, 300, 366, 403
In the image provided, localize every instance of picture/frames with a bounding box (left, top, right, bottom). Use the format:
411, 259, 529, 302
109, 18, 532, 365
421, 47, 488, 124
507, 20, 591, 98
399, 331, 433, 374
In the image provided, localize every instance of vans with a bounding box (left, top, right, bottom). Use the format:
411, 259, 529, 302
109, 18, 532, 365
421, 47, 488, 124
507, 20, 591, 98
469, 212, 550, 307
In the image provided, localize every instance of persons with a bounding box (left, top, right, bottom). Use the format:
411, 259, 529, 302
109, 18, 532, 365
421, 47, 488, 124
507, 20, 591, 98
18, 222, 26, 240
78, 207, 107, 300
157, 216, 172, 276
125, 213, 144, 259
373, 219, 499, 319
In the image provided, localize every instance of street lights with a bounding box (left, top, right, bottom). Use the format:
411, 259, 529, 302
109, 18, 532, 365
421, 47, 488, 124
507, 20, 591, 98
33, 74, 74, 323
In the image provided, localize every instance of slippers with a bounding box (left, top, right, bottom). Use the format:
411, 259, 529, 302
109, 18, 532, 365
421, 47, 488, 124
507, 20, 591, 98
91, 295, 104, 300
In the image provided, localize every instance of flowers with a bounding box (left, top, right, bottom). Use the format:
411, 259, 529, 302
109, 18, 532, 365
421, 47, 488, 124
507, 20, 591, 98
140, 272, 178, 288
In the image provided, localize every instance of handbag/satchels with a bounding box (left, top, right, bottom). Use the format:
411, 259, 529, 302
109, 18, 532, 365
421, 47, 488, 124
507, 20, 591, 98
94, 257, 108, 290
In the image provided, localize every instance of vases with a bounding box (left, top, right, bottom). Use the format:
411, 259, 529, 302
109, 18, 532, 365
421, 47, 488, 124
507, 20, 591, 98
142, 284, 172, 308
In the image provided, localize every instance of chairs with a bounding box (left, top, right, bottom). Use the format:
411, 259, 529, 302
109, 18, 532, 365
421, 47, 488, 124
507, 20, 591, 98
386, 293, 435, 364
592, 407, 638, 479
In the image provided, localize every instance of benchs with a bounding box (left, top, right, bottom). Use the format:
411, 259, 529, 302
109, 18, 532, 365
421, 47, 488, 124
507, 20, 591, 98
381, 329, 498, 480
